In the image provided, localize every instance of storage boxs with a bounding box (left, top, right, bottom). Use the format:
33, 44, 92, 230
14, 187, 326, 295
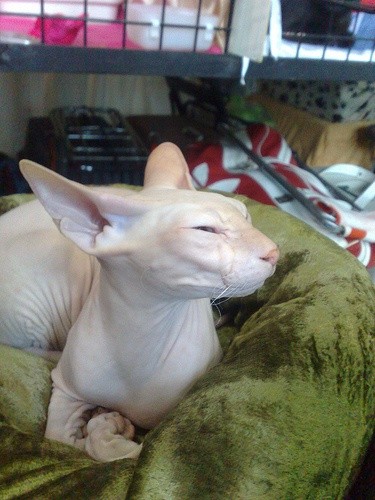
245, 89, 375, 180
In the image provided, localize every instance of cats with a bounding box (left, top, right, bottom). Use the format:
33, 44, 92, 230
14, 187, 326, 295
1, 141, 280, 463
